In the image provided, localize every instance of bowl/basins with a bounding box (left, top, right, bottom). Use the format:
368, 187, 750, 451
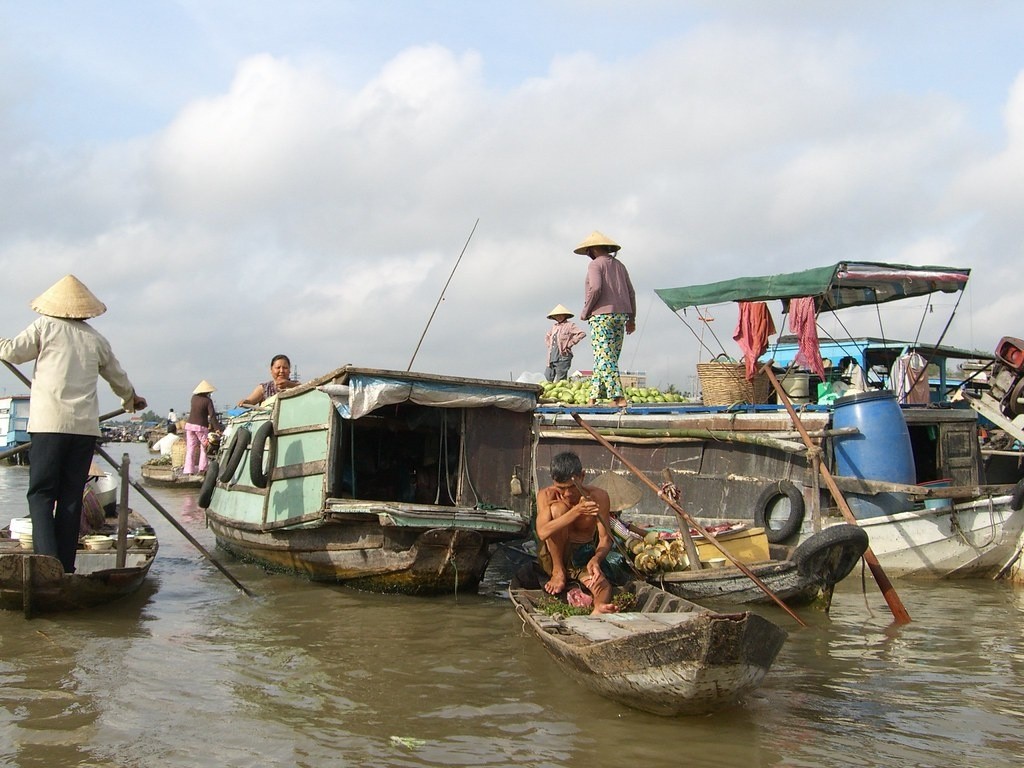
81, 534, 156, 549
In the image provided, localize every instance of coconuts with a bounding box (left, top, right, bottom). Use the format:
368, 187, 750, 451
537, 380, 692, 405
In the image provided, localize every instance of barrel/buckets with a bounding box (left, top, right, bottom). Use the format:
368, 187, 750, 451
9, 517, 33, 550
775, 374, 810, 412
832, 389, 916, 519
917, 478, 955, 510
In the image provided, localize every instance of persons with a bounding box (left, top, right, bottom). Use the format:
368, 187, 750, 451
167, 409, 176, 424
545, 305, 586, 385
152, 425, 179, 458
53, 458, 107, 534
573, 230, 637, 409
0, 274, 144, 570
536, 450, 622, 615
236, 355, 301, 409
183, 379, 220, 475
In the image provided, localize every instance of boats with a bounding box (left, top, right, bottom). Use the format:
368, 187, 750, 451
525, 260, 1024, 581
0, 392, 33, 464
197, 361, 550, 593
495, 559, 790, 722
639, 519, 873, 606
616, 521, 776, 568
142, 425, 170, 454
139, 459, 206, 492
0, 451, 164, 619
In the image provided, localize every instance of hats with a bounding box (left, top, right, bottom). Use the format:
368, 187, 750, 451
587, 469, 643, 512
573, 232, 621, 255
546, 304, 574, 320
32, 275, 107, 318
88, 460, 107, 477
193, 380, 217, 395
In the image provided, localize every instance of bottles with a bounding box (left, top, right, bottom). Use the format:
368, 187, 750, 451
511, 474, 522, 494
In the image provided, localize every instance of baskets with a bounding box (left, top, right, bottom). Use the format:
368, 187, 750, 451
172, 437, 200, 467
696, 353, 771, 407
